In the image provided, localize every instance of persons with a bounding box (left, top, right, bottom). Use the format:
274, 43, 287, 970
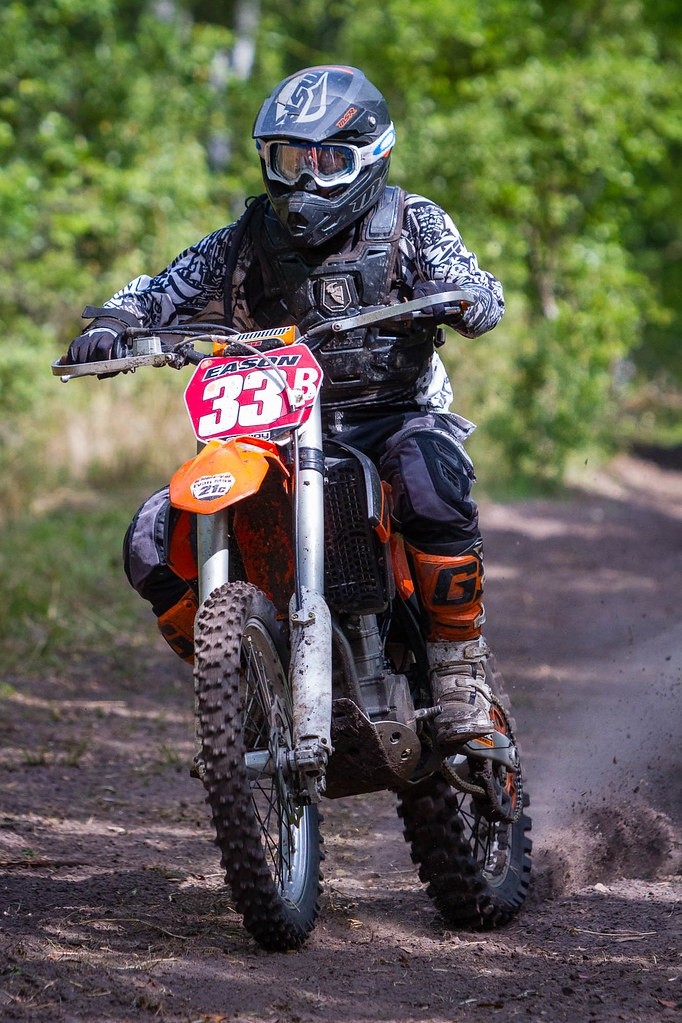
66, 63, 504, 779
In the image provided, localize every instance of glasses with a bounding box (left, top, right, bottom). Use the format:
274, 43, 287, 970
263, 140, 363, 187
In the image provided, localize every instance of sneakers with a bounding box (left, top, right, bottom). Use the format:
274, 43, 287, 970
426, 638, 498, 740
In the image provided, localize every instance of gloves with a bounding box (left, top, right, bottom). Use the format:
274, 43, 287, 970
67, 319, 131, 379
411, 281, 465, 326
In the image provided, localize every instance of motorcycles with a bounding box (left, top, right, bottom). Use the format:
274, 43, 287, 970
52, 289, 536, 951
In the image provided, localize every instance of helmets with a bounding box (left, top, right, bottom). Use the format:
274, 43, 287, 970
252, 64, 395, 247
